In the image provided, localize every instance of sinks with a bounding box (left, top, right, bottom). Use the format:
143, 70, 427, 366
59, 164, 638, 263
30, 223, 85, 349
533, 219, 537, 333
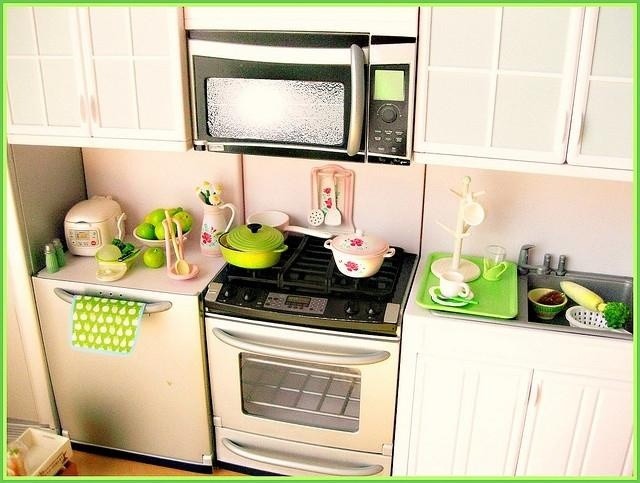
518, 267, 634, 338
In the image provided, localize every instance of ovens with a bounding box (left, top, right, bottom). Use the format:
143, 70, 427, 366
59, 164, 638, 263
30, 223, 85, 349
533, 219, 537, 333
204, 315, 400, 457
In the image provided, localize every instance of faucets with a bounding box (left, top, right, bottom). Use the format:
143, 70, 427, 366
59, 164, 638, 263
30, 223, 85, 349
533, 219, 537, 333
523, 262, 550, 277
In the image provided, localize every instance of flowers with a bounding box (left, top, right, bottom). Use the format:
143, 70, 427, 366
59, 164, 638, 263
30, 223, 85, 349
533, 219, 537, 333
195, 179, 225, 205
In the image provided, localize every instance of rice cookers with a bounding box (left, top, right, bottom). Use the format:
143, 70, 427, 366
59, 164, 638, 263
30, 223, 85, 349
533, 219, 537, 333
65, 194, 125, 256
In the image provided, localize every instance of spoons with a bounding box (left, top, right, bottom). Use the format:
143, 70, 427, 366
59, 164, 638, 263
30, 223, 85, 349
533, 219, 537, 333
165, 209, 190, 274
438, 293, 479, 304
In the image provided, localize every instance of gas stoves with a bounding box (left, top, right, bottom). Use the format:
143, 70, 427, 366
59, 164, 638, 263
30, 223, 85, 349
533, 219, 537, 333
201, 236, 419, 337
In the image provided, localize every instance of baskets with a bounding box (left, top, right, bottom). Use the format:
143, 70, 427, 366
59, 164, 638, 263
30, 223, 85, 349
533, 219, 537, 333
566, 305, 633, 336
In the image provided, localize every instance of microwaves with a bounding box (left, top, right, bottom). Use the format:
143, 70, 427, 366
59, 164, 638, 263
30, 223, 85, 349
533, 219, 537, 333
183, 29, 417, 169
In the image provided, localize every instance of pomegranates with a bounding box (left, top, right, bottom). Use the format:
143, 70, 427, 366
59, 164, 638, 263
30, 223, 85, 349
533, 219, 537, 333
143, 248, 164, 268
136, 207, 193, 240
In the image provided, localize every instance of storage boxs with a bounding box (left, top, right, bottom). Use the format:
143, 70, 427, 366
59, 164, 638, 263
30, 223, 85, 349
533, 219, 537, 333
9, 428, 74, 476
57, 461, 78, 476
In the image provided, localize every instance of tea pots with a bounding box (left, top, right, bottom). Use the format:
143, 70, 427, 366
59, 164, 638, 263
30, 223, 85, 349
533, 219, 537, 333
200, 200, 236, 260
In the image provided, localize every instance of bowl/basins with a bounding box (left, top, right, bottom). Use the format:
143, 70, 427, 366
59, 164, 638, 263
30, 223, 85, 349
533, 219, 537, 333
527, 287, 568, 320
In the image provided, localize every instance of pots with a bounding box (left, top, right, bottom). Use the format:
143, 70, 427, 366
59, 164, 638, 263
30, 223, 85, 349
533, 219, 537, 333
323, 230, 396, 278
217, 223, 288, 268
247, 209, 334, 239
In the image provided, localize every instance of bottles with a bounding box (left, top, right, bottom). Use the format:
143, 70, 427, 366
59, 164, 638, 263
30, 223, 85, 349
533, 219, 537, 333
45, 239, 66, 273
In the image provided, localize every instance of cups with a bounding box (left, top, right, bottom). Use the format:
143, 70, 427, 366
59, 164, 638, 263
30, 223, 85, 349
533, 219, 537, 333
462, 190, 488, 226
482, 245, 508, 282
440, 271, 469, 298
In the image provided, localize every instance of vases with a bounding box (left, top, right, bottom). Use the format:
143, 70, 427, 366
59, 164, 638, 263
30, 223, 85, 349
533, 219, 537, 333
199, 191, 237, 258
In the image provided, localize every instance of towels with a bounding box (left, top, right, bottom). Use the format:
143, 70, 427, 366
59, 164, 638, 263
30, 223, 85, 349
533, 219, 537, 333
68, 291, 146, 356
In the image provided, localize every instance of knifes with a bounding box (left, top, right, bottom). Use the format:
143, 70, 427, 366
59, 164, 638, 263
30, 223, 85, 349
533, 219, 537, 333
117, 247, 141, 263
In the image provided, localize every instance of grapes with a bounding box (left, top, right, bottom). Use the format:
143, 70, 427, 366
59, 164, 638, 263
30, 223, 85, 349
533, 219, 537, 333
537, 290, 563, 305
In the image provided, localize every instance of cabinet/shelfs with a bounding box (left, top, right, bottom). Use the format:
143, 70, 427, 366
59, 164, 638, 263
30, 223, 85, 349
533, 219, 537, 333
3, 3, 193, 152
7, 142, 89, 431
413, 3, 634, 181
393, 309, 633, 478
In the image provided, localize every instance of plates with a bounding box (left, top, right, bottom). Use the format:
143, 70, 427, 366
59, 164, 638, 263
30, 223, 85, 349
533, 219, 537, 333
132, 224, 193, 248
429, 285, 475, 306
94, 250, 139, 263
